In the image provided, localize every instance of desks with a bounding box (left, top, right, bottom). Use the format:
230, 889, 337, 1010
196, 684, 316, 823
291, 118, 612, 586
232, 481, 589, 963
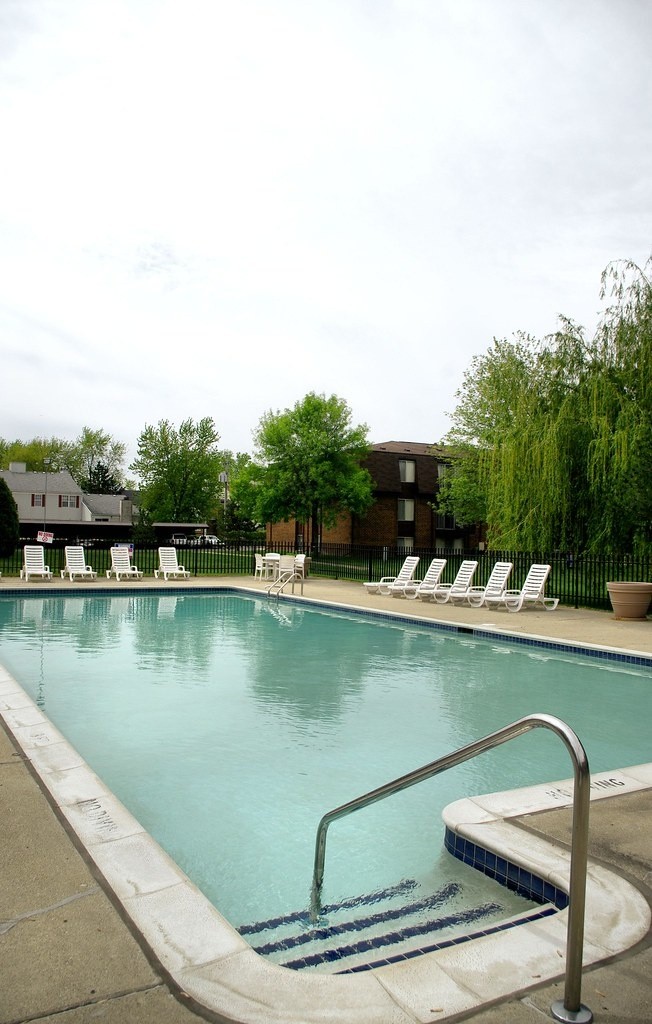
262, 556, 298, 580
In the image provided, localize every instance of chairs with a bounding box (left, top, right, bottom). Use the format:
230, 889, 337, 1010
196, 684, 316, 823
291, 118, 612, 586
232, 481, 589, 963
60, 546, 97, 582
484, 564, 559, 612
154, 547, 190, 581
447, 562, 513, 610
417, 560, 478, 606
363, 557, 420, 597
388, 559, 447, 602
106, 547, 143, 581
254, 553, 312, 582
20, 545, 53, 582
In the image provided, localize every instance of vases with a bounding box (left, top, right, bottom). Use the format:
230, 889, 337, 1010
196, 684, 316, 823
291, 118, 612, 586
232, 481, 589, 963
606, 581, 652, 617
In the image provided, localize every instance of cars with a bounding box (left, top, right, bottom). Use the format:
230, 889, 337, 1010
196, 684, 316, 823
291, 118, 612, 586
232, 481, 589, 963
165, 533, 187, 544
187, 535, 225, 545
